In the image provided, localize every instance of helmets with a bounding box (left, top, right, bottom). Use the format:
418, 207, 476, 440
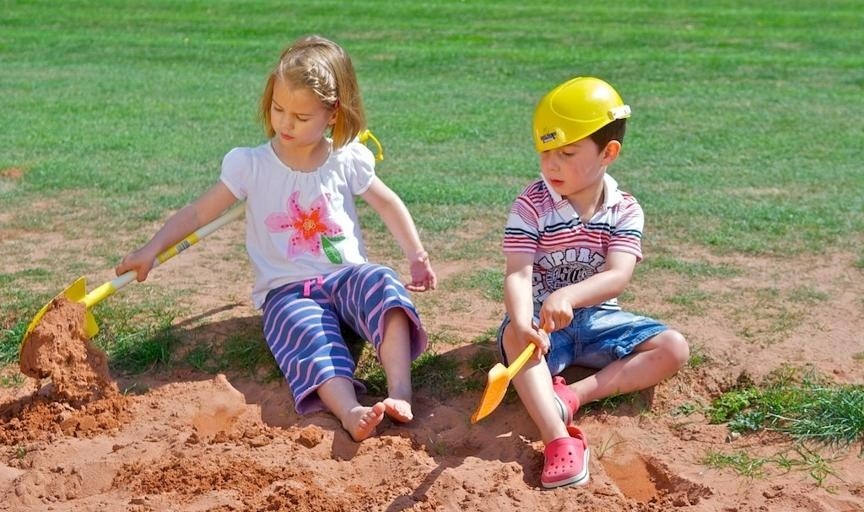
532, 77, 631, 153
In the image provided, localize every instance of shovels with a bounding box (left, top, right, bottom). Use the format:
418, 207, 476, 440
18, 128, 383, 378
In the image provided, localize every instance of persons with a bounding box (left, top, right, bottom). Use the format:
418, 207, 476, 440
494, 76, 692, 488
114, 33, 434, 441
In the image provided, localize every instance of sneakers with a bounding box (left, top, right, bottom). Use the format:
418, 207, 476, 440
540, 426, 590, 490
553, 376, 580, 425
